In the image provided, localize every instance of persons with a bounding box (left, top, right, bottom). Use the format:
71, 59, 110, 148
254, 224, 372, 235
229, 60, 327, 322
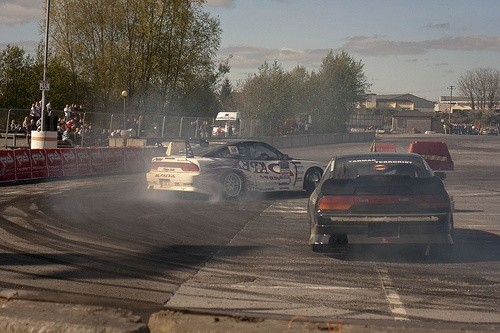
273, 121, 307, 136
191, 119, 233, 140
57, 103, 122, 147
129, 117, 140, 137
365, 125, 375, 131
10, 97, 52, 138
442, 120, 500, 135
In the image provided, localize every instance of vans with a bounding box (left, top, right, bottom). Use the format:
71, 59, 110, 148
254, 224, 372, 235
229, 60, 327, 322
210, 111, 245, 139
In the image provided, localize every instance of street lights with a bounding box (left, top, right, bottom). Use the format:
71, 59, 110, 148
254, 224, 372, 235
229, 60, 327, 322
121, 90, 128, 147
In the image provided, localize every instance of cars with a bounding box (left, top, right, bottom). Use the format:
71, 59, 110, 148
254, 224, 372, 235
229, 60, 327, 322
307, 151, 456, 259
145, 137, 328, 200
